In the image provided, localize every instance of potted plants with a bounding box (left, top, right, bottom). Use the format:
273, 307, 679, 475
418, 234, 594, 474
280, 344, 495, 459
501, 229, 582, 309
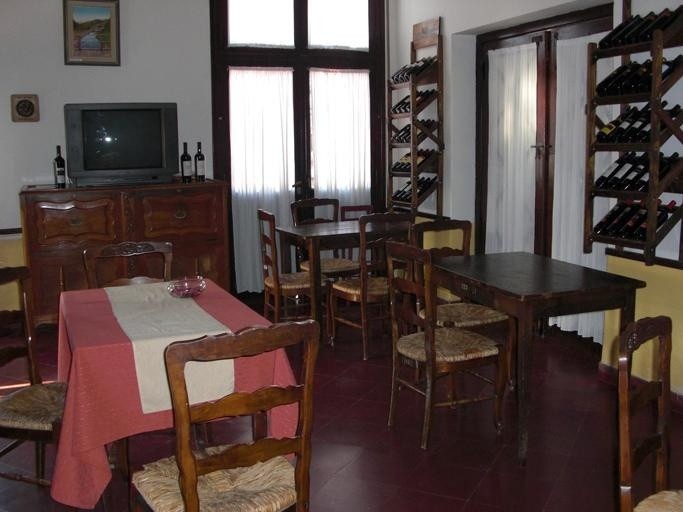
62, 0, 121, 66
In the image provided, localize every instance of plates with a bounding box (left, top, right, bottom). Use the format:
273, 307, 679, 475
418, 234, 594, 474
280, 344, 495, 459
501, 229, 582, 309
387, 15, 444, 225
584, 5, 683, 269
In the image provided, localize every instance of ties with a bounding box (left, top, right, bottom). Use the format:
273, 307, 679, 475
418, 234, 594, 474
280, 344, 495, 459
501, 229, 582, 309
64, 102, 181, 187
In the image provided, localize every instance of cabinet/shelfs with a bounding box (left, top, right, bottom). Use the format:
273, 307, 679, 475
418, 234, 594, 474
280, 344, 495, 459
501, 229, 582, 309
584, 5, 683, 269
387, 15, 444, 225
194, 142, 206, 184
389, 56, 436, 203
594, 8, 681, 243
52, 145, 65, 190
180, 142, 192, 184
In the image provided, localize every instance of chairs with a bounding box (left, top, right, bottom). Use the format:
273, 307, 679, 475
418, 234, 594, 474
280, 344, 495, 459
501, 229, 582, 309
92, 241, 173, 287
385, 241, 509, 451
130, 319, 321, 511
0, 267, 133, 511
618, 315, 683, 511
409, 219, 518, 404
258, 199, 434, 362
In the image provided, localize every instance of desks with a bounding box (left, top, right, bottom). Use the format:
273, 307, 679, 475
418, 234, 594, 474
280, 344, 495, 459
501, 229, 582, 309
57, 278, 303, 510
422, 251, 647, 466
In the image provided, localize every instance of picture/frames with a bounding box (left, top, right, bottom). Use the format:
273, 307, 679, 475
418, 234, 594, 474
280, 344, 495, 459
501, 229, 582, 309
62, 0, 121, 66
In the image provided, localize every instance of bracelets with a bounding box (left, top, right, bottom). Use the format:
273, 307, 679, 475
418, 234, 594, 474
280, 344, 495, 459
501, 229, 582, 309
167, 275, 206, 299
